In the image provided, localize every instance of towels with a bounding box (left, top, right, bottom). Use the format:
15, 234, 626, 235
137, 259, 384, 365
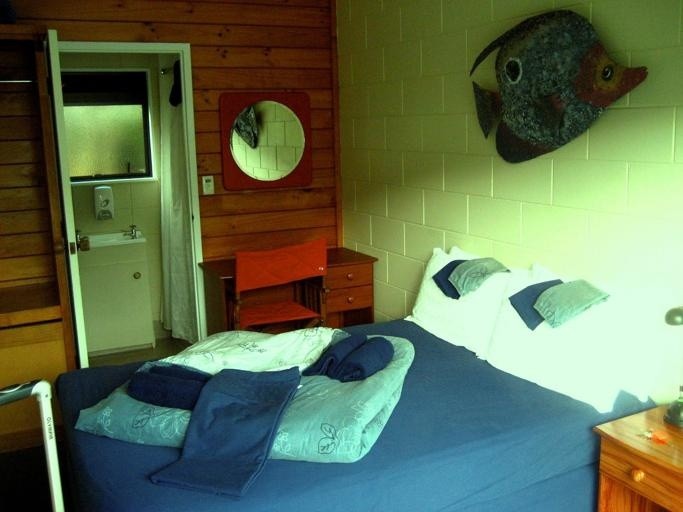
129, 358, 213, 411
148, 367, 304, 494
302, 333, 394, 382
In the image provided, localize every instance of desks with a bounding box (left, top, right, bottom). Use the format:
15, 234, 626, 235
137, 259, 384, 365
197, 247, 379, 338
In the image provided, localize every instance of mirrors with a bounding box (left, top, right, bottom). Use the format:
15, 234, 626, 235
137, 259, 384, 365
217, 89, 313, 191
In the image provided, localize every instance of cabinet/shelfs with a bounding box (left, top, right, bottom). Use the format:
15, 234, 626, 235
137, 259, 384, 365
79, 246, 156, 359
0, 34, 78, 454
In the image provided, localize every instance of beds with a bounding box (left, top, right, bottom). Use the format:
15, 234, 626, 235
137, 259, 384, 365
52, 244, 656, 512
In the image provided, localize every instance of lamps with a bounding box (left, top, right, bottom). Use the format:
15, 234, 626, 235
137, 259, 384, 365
663, 304, 683, 427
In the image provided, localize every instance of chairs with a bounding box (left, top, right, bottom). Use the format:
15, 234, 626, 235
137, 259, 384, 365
229, 237, 328, 336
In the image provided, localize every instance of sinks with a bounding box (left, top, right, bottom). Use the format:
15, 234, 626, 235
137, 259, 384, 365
77, 230, 146, 246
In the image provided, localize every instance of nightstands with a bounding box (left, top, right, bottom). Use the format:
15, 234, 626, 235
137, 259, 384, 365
591, 403, 682, 512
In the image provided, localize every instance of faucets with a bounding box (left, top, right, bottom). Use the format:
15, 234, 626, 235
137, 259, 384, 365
125, 224, 138, 239
